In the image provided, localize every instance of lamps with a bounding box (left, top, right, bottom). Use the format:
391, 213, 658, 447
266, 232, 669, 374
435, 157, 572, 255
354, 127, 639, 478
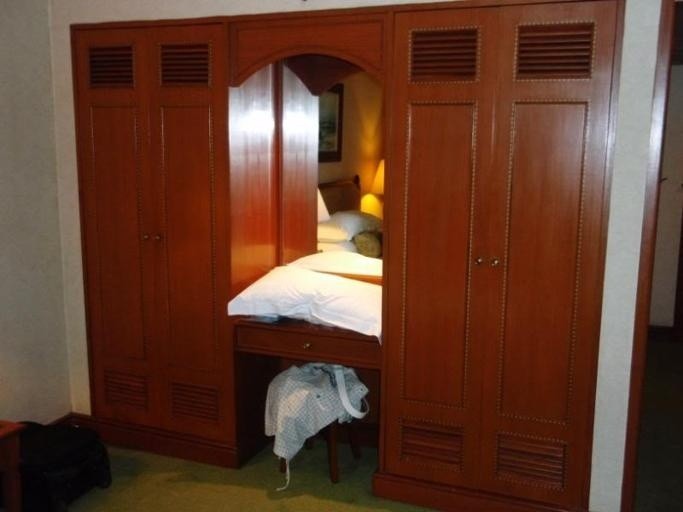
371, 159, 384, 196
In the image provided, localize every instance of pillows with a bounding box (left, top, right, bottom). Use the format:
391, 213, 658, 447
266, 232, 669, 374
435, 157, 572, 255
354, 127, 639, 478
226, 266, 383, 345
287, 249, 382, 276
354, 228, 380, 258
317, 208, 381, 242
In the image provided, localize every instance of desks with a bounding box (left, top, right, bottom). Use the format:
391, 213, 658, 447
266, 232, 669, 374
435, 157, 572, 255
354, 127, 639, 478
0, 419, 29, 510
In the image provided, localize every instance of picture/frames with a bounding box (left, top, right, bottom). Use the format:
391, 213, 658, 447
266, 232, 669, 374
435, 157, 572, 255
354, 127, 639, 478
318, 82, 344, 163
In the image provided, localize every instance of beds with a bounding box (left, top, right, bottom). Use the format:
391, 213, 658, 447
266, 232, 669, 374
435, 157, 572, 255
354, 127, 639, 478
317, 174, 381, 258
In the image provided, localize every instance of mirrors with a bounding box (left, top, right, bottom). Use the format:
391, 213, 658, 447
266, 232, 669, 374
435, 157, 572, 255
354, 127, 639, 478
276, 53, 383, 277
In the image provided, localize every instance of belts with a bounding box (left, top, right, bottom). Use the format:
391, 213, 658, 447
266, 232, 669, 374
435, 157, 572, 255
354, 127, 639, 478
332, 365, 370, 419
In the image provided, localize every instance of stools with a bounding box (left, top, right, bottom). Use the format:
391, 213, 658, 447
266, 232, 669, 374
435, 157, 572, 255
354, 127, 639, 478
265, 362, 363, 483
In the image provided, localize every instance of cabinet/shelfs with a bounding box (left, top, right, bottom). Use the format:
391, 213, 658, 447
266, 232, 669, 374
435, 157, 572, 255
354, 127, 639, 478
230, 9, 388, 370
369, 0, 625, 511
67, 16, 240, 468
